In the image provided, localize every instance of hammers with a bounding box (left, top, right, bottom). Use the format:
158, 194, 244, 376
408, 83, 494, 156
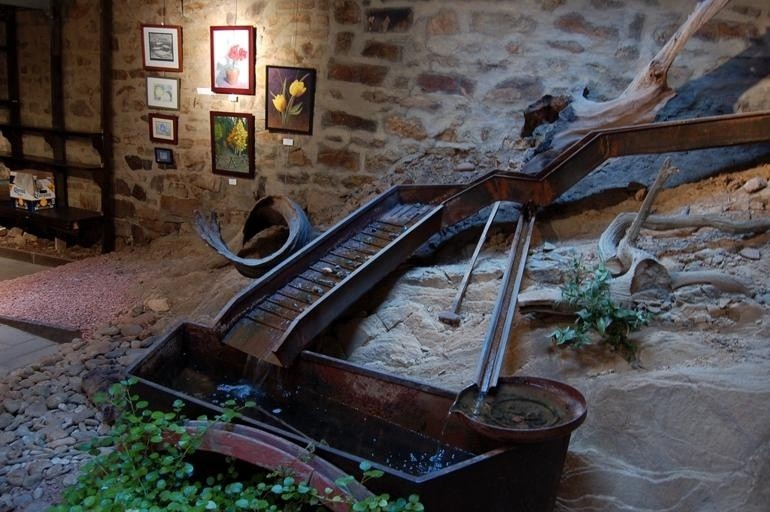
438, 201, 499, 323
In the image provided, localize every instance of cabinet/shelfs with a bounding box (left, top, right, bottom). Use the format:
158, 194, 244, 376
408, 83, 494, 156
0, 0, 114, 256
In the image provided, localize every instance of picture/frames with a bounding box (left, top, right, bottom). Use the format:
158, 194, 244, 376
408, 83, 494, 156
140, 23, 317, 181
